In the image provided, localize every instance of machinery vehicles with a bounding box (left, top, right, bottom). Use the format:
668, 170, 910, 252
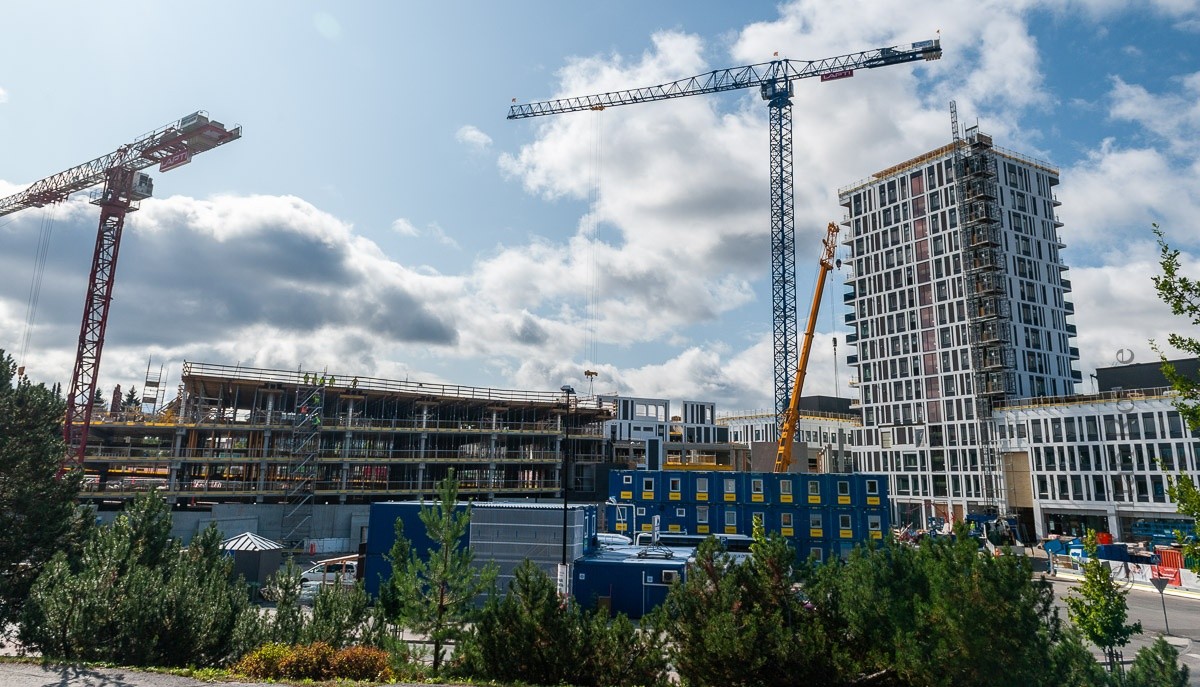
769, 222, 845, 478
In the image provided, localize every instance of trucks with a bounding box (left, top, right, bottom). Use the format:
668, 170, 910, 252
978, 518, 1026, 557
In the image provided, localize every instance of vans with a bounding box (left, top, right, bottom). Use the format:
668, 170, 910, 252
299, 560, 358, 587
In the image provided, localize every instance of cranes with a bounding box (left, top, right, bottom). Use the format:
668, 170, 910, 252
506, 35, 946, 443
0, 106, 242, 495
